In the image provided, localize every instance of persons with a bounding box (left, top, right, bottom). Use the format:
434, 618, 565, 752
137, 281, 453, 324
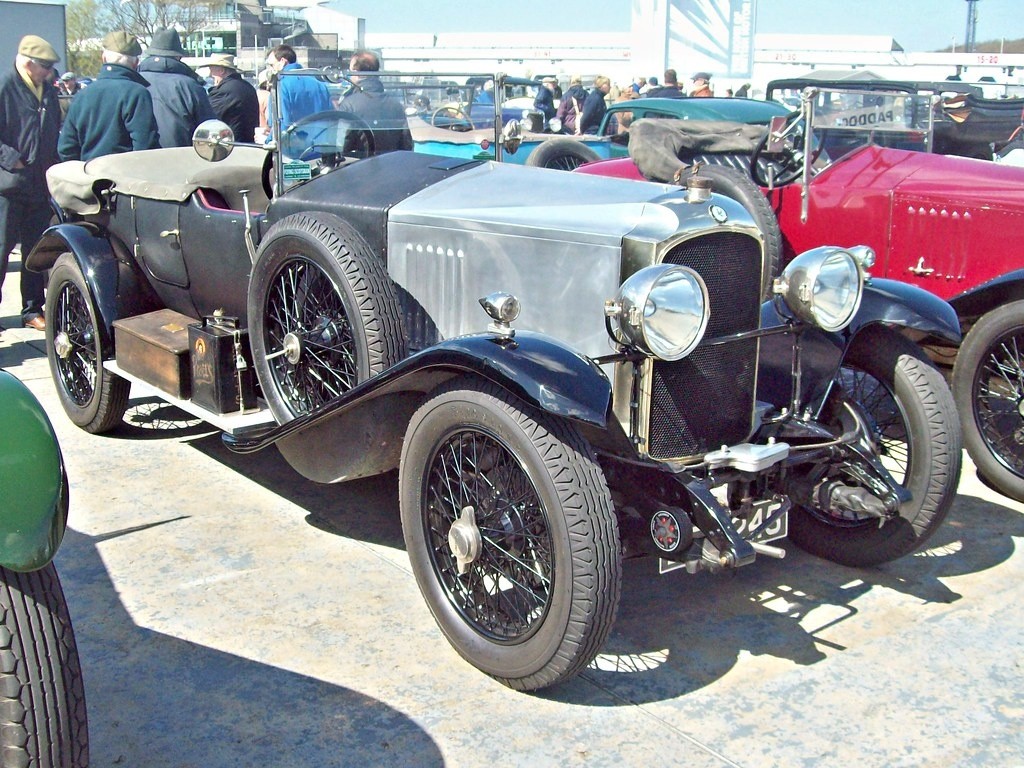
413, 62, 751, 137
0, 27, 414, 331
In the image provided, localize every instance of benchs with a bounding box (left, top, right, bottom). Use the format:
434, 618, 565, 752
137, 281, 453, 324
627, 118, 797, 187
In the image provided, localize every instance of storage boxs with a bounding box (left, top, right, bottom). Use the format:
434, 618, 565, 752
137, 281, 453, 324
112, 309, 202, 401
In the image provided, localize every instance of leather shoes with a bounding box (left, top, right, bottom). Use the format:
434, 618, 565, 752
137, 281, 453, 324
22, 316, 46, 330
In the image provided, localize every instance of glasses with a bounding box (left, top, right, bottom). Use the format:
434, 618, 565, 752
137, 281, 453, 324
31, 58, 54, 71
693, 80, 696, 84
63, 80, 70, 82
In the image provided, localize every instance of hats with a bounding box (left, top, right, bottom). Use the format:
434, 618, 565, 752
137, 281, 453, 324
542, 77, 554, 83
18, 35, 61, 63
690, 72, 713, 80
144, 27, 185, 56
198, 52, 241, 70
103, 31, 143, 55
61, 72, 75, 80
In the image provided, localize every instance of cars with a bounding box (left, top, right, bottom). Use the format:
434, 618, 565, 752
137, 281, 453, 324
33, 65, 962, 690
415, 48, 1023, 520
1, 367, 99, 768
